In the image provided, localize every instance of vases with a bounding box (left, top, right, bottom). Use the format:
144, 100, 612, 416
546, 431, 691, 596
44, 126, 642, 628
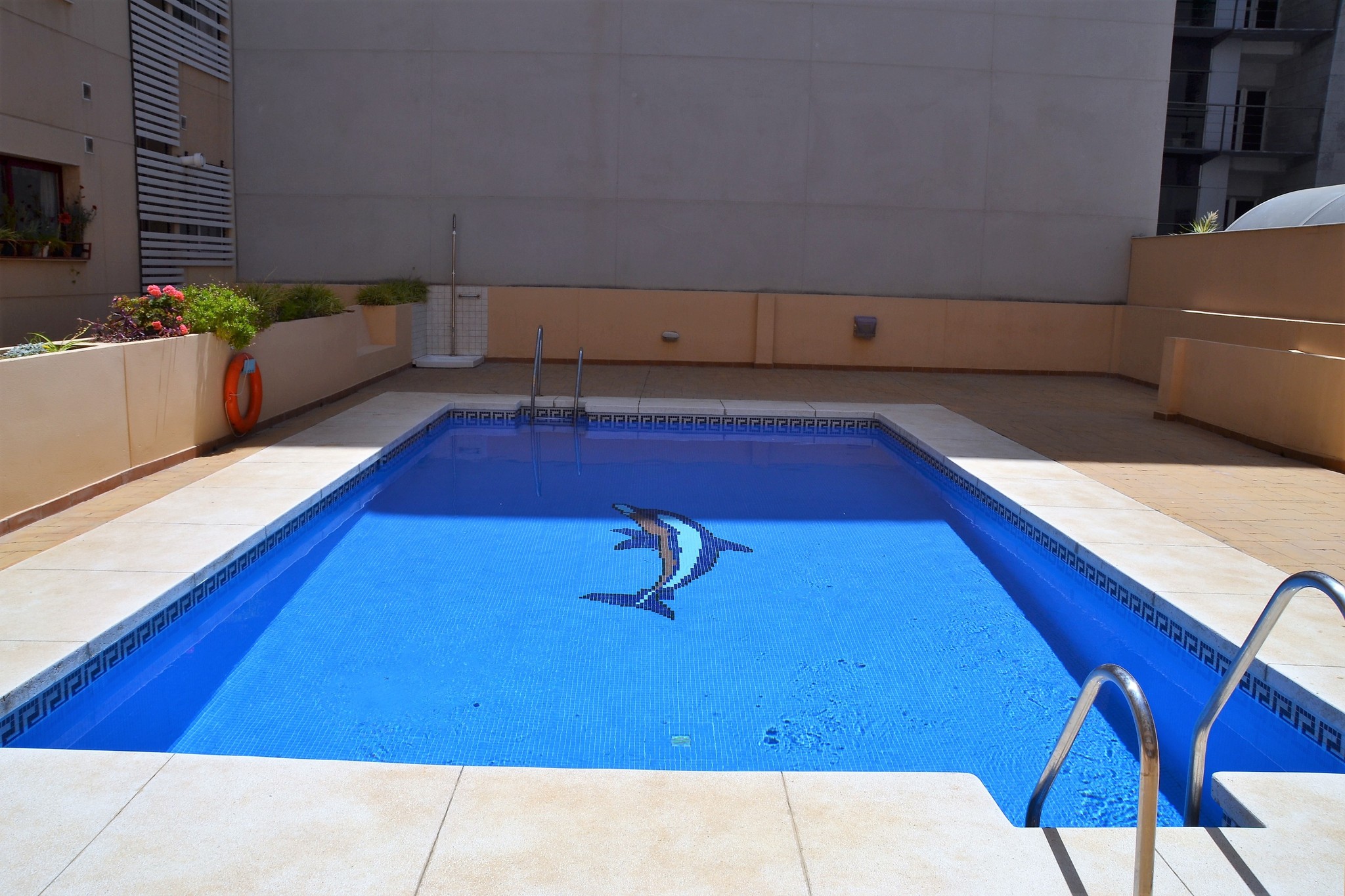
72, 243, 84, 257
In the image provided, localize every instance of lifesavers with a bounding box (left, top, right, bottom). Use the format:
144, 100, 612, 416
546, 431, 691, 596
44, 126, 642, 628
226, 351, 264, 434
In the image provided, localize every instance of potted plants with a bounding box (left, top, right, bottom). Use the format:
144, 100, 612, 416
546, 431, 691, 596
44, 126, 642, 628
0, 192, 70, 257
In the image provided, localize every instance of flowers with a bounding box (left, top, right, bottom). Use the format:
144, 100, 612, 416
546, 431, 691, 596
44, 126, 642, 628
50, 182, 98, 242
